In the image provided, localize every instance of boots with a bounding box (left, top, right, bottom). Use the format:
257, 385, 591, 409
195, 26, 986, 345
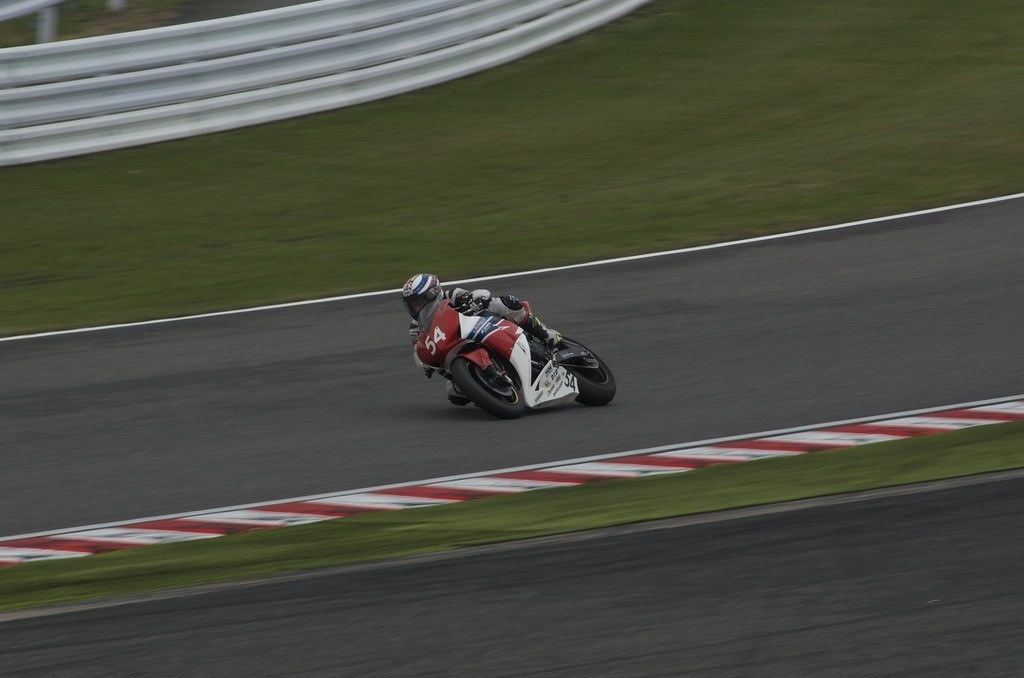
531, 317, 562, 347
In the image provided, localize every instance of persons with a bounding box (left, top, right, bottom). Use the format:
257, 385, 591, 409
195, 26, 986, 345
404, 274, 561, 406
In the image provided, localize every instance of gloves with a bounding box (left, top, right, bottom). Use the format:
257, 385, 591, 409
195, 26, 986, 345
468, 297, 486, 311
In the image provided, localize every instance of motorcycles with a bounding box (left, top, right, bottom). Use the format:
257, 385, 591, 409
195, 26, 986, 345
410, 297, 616, 420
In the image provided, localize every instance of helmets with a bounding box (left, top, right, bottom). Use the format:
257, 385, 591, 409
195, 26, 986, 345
402, 273, 444, 319
